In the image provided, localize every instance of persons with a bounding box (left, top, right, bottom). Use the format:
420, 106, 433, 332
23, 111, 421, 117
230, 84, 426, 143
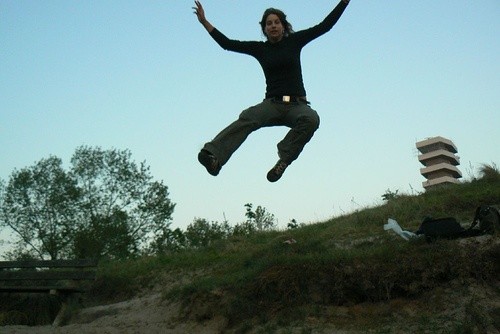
192, 1, 351, 182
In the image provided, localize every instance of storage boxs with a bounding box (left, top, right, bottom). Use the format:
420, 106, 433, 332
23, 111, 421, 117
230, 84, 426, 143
415, 136, 463, 190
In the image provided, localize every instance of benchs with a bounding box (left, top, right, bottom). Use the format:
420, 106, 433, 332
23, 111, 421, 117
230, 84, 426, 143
1, 259, 98, 315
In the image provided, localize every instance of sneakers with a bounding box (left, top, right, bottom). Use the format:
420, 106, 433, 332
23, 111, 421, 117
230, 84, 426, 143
267, 159, 290, 182
198, 151, 221, 176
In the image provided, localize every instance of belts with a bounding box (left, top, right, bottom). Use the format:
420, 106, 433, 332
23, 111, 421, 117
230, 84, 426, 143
267, 95, 306, 103
